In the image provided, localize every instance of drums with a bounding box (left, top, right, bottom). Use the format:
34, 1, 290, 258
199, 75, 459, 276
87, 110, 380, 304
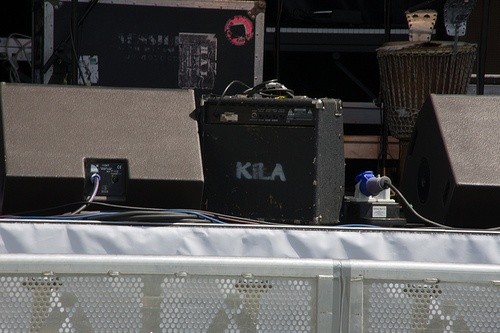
378, 39, 478, 140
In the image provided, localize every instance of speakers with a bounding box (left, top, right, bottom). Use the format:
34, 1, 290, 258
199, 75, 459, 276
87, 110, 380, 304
0, 81, 204, 217
197, 99, 344, 225
398, 93, 500, 230
